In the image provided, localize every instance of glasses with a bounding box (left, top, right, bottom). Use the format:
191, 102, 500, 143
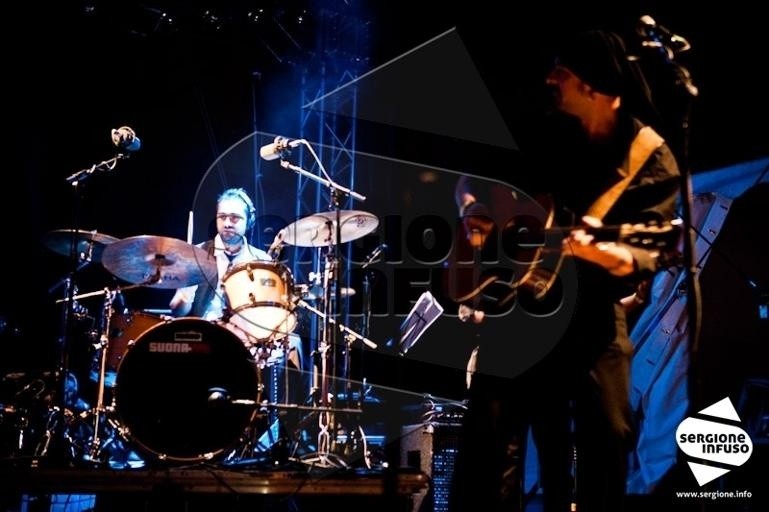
216, 214, 245, 224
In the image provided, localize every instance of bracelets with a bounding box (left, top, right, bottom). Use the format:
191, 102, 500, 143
608, 244, 633, 278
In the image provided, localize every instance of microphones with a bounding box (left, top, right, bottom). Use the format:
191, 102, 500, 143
359, 243, 388, 269
209, 389, 229, 407
117, 287, 133, 325
635, 15, 686, 46
112, 126, 141, 151
291, 295, 308, 309
260, 136, 295, 161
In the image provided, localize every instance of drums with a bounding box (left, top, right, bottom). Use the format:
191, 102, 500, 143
114, 313, 264, 461
222, 261, 298, 345
106, 312, 168, 370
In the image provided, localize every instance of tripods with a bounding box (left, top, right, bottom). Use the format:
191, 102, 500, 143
264, 140, 375, 466
74, 309, 129, 459
277, 343, 312, 457
226, 348, 274, 463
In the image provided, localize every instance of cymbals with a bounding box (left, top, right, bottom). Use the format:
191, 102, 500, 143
103, 235, 218, 289
44, 229, 121, 263
281, 210, 379, 247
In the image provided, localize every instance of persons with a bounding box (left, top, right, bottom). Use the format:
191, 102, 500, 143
462, 201, 494, 249
449, 29, 683, 512
168, 187, 273, 321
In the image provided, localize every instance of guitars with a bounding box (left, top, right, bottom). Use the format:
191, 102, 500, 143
447, 185, 683, 310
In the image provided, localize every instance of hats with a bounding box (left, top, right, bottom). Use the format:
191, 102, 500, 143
560, 22, 654, 106
